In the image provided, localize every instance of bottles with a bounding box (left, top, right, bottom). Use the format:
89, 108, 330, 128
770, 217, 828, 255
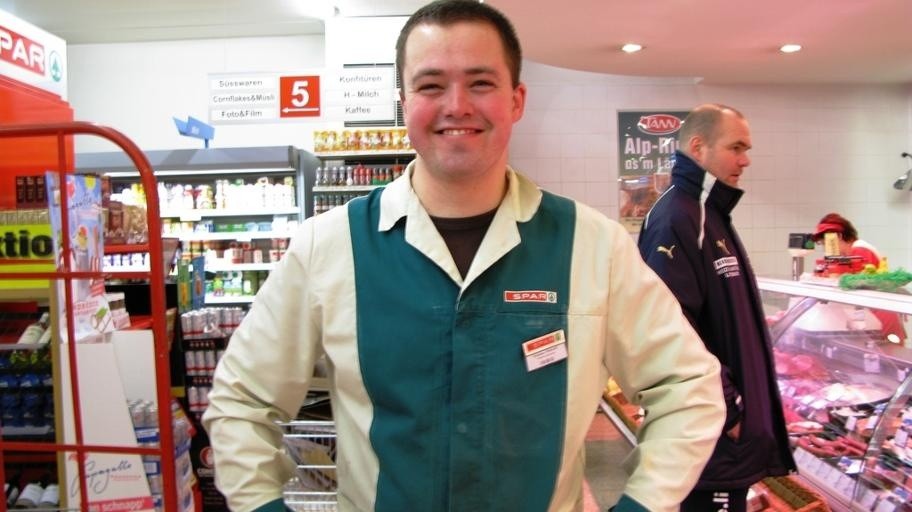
0, 312, 57, 509
213, 275, 224, 296
182, 184, 193, 207
313, 164, 405, 216
214, 179, 227, 210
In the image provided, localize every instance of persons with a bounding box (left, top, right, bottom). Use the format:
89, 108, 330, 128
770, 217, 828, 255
811, 212, 908, 347
638, 102, 798, 510
198, 1, 729, 511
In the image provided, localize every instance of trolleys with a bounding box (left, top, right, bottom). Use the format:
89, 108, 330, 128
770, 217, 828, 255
275, 393, 339, 510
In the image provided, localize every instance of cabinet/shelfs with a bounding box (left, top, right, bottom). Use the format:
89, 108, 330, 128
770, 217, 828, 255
753, 275, 911, 510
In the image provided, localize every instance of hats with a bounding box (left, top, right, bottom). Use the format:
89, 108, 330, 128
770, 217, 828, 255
811, 222, 844, 242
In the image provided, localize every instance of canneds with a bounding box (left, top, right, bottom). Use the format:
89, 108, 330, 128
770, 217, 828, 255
180, 306, 242, 413
